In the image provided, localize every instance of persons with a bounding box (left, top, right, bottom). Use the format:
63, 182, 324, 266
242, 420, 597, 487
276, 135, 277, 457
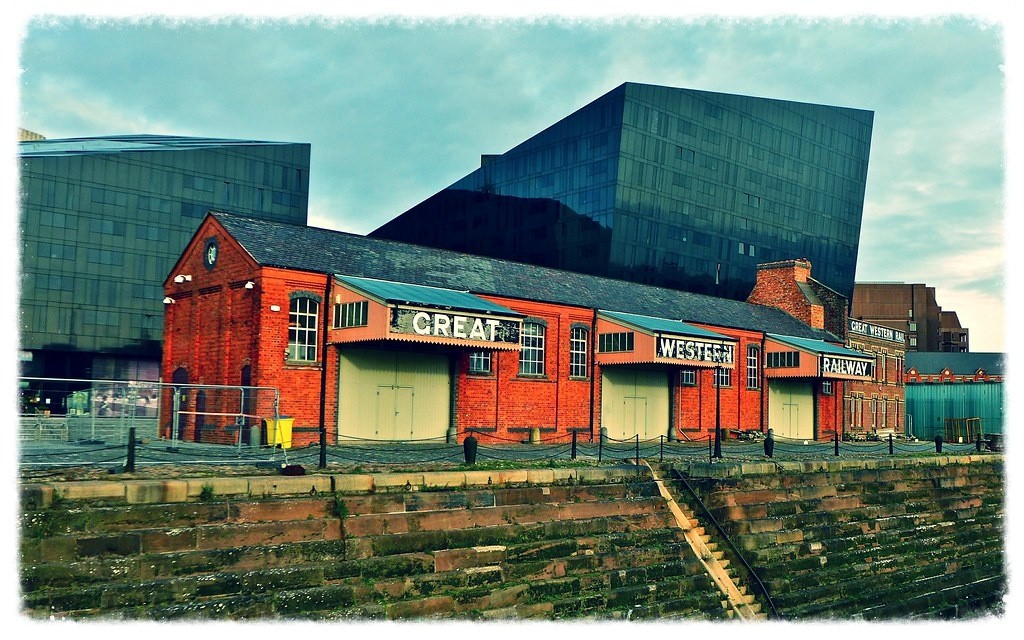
98, 395, 113, 415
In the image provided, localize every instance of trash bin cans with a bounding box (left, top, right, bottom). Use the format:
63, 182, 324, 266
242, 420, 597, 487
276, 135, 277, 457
263, 415, 295, 448
